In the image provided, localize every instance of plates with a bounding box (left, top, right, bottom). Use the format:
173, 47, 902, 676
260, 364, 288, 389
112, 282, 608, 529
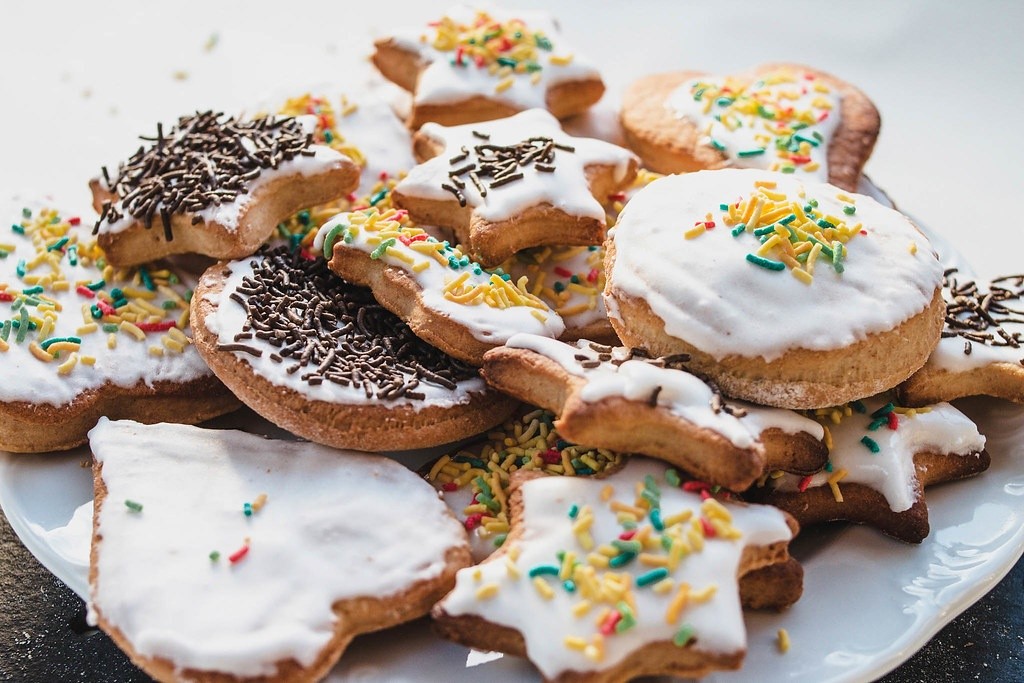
0, 258, 1024, 683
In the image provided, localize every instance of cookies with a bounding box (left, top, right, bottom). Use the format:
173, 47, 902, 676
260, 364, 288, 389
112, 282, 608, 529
0, 13, 1024, 683
